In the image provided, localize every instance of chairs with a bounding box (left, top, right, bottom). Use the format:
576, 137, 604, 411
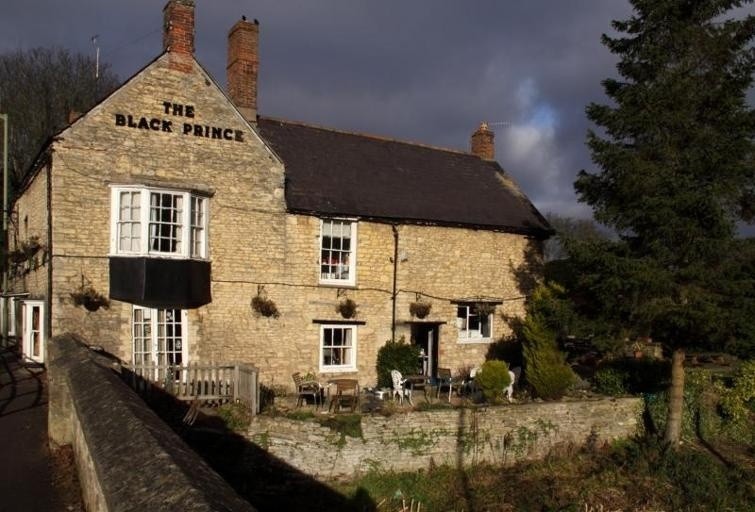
390, 367, 482, 407
291, 372, 362, 413
